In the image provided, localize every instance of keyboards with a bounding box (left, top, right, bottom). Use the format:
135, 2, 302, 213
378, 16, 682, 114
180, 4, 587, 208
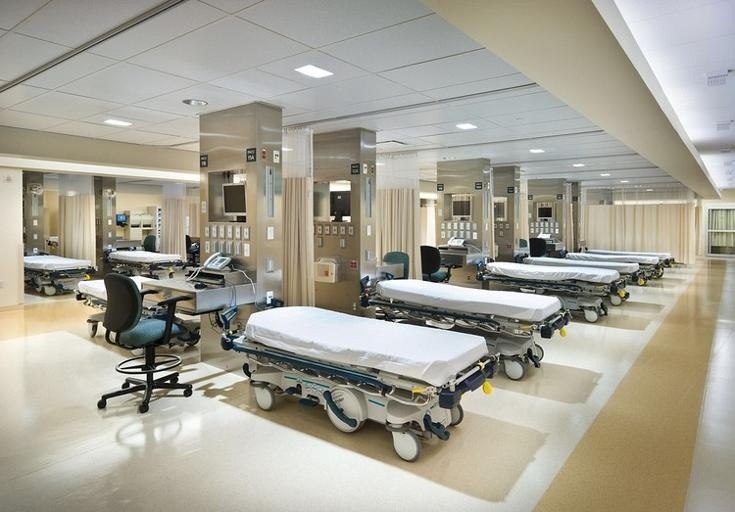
190, 276, 224, 285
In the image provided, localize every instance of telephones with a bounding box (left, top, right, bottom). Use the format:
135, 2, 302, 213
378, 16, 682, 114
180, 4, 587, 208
537, 233, 551, 239
448, 237, 465, 246
204, 252, 231, 270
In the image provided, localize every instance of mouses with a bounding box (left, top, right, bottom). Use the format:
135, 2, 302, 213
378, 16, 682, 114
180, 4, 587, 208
195, 283, 207, 289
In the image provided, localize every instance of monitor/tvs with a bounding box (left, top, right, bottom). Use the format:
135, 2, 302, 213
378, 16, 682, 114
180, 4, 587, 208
330, 191, 350, 222
221, 182, 246, 222
538, 207, 552, 221
117, 214, 127, 223
453, 200, 470, 220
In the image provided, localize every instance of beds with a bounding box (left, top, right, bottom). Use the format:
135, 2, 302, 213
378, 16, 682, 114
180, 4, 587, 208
229, 296, 493, 466
21, 247, 200, 330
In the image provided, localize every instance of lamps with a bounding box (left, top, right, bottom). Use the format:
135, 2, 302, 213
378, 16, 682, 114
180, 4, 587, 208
570, 162, 658, 194
455, 119, 481, 134
102, 115, 134, 134
293, 65, 336, 81
528, 147, 546, 159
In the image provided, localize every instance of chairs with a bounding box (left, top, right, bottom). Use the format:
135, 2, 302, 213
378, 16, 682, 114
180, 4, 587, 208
362, 248, 410, 319
94, 273, 193, 416
183, 232, 202, 270
419, 244, 456, 283
530, 238, 547, 257
139, 233, 158, 254
